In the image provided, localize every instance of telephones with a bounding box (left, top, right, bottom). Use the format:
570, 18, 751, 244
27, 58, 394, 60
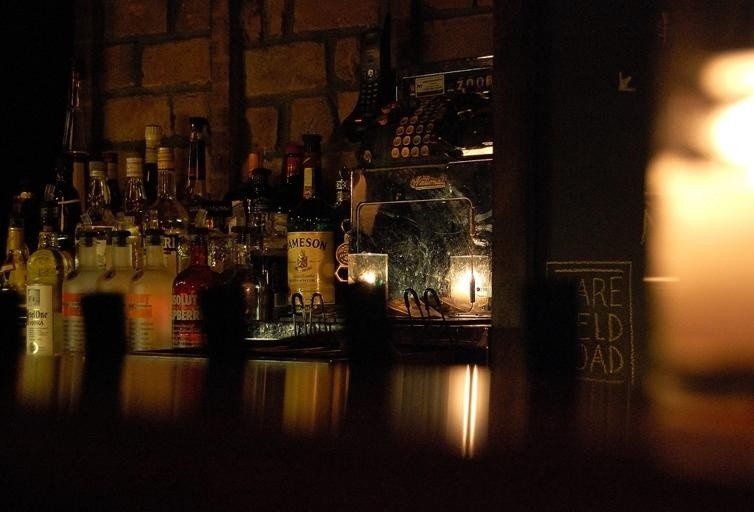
341, 13, 388, 127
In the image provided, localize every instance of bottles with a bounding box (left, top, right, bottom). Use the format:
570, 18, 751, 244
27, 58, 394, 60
5, 117, 329, 356
